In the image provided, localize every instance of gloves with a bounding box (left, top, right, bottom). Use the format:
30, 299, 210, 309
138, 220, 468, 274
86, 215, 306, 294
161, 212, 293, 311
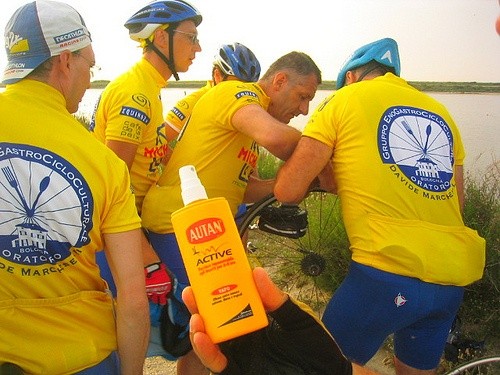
144, 262, 178, 306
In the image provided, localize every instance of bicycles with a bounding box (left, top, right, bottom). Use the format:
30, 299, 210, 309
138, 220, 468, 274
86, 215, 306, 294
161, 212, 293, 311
445, 356, 500, 374
238, 181, 352, 322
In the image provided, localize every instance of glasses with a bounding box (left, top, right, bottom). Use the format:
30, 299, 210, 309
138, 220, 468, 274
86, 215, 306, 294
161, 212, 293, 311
165, 29, 199, 45
72, 52, 101, 83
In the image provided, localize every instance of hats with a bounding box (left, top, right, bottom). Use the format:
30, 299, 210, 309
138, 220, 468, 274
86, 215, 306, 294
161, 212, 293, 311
0, 0, 92, 88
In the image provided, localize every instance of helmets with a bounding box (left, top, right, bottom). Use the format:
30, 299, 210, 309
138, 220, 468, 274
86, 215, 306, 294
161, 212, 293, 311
123, 0, 202, 42
212, 42, 261, 82
336, 38, 400, 90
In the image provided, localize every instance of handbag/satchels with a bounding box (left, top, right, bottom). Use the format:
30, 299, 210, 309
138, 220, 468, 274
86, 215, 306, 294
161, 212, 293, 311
445, 332, 485, 364
258, 205, 308, 239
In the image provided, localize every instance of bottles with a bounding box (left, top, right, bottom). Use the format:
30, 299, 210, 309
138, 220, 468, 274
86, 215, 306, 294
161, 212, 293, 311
170, 165, 268, 344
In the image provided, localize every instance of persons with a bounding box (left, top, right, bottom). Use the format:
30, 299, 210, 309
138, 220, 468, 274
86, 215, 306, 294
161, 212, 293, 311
141, 48, 322, 352
161, 39, 262, 163
274, 36, 486, 375
89, 0, 203, 348
0, 0, 150, 373
176, 263, 355, 369
